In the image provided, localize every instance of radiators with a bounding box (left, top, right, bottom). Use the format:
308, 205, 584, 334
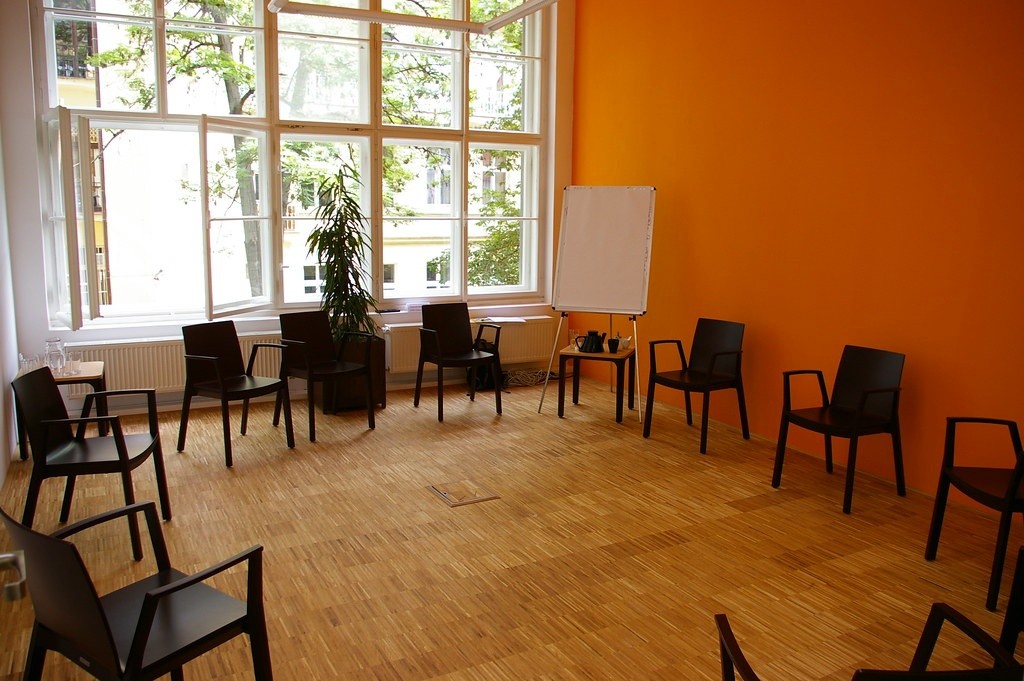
70, 330, 282, 400
386, 315, 555, 375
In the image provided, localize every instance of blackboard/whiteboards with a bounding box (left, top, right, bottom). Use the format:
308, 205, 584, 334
552, 185, 656, 316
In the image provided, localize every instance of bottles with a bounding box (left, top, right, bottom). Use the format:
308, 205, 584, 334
614, 331, 621, 350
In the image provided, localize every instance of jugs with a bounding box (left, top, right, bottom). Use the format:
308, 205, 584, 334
575, 331, 606, 353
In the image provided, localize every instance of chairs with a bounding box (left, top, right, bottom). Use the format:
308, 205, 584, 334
413, 302, 502, 423
0, 500, 273, 681
770, 345, 905, 516
713, 600, 1024, 681
10, 366, 172, 562
924, 417, 1024, 612
642, 317, 749, 454
272, 311, 375, 443
176, 320, 295, 467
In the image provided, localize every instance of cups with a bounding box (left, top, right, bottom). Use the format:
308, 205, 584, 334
68, 351, 82, 374
622, 339, 630, 348
18, 351, 39, 374
607, 339, 620, 354
568, 329, 579, 350
43, 339, 66, 373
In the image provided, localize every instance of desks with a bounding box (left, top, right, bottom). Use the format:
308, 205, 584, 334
559, 342, 635, 423
15, 361, 110, 461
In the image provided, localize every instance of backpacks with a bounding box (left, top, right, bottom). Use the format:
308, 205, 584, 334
466, 338, 510, 392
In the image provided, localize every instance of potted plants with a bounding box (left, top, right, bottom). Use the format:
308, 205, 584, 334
304, 144, 387, 415
